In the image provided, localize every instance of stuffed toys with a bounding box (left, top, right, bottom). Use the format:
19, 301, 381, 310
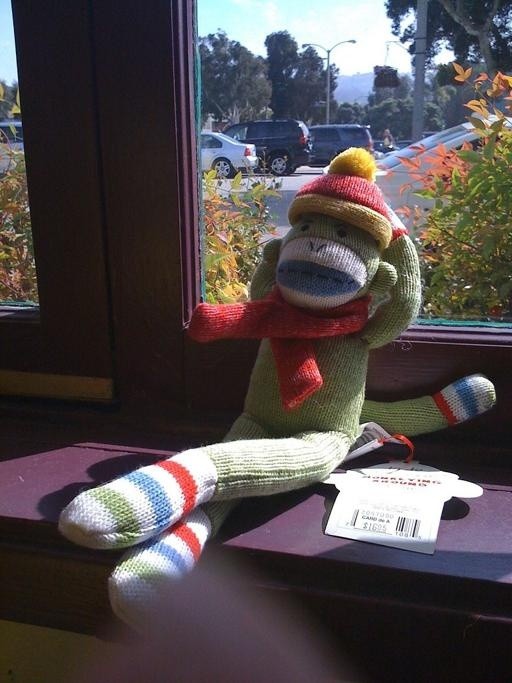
58, 149, 496, 632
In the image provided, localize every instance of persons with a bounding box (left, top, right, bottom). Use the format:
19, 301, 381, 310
381, 129, 395, 152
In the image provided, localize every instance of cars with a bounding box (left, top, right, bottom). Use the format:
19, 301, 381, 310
0, 121, 24, 172
200, 118, 373, 180
375, 114, 512, 250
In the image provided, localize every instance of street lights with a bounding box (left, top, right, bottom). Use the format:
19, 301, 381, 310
302, 40, 356, 123
